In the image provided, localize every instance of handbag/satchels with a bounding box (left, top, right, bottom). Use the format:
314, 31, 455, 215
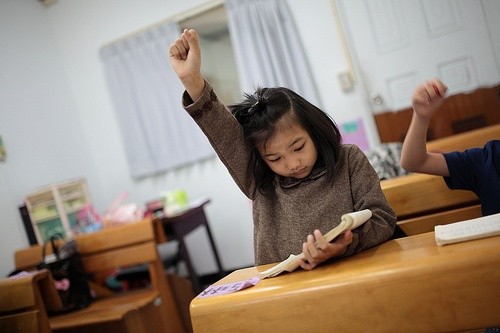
37, 232, 92, 312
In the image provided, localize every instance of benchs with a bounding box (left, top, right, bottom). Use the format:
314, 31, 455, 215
381, 172, 484, 239
0, 266, 64, 333
13, 218, 186, 332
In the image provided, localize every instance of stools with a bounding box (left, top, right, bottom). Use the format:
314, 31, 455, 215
114, 253, 181, 292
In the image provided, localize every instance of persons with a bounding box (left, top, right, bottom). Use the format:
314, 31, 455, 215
169, 28, 397, 271
401, 80, 500, 216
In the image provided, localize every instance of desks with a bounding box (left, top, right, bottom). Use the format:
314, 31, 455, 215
189, 211, 500, 333
145, 199, 226, 296
426, 123, 500, 154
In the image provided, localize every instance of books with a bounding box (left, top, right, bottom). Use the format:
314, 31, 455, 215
434, 213, 497, 247
261, 208, 372, 279
